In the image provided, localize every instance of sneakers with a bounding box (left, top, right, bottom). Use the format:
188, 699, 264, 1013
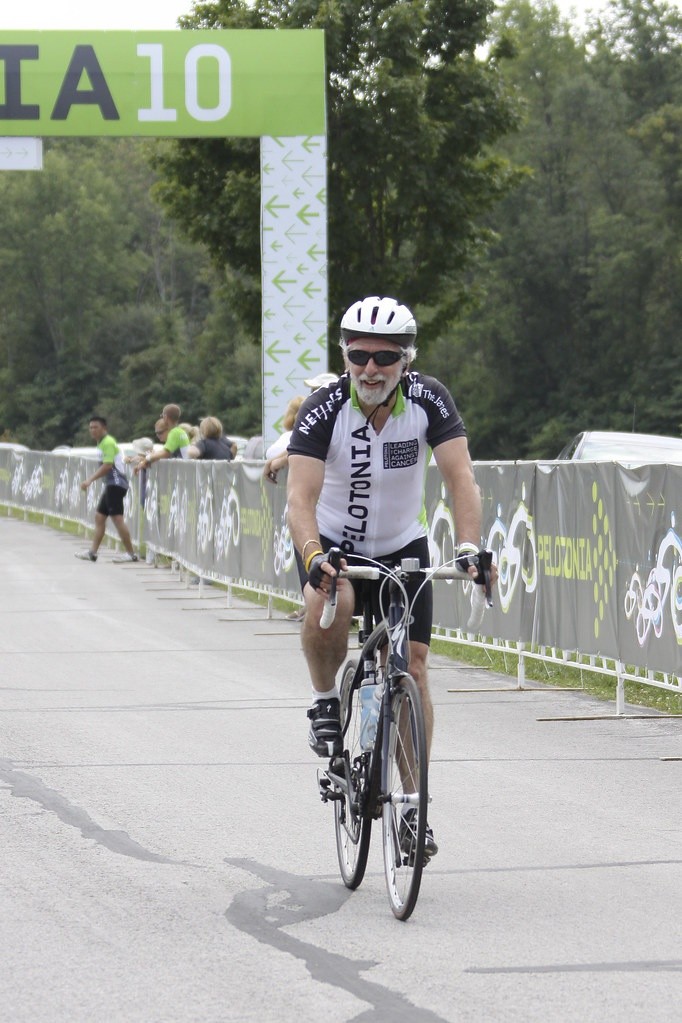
112, 552, 139, 563
400, 808, 439, 856
306, 698, 344, 758
74, 549, 97, 562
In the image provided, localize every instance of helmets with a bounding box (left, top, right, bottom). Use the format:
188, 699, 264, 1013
340, 296, 417, 348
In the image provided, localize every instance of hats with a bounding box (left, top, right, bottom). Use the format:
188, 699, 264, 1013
304, 373, 339, 387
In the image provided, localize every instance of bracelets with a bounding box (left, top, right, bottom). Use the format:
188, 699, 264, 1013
302, 540, 324, 573
456, 542, 480, 556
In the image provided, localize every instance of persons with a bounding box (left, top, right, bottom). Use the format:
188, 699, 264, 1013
264, 373, 340, 485
74, 416, 137, 563
286, 296, 499, 855
124, 404, 237, 475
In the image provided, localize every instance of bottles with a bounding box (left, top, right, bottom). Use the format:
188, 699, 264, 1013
358, 676, 386, 752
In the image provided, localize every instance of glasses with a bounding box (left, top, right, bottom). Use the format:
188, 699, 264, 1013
347, 349, 405, 366
155, 429, 166, 435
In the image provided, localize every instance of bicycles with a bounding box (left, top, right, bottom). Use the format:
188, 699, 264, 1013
299, 545, 495, 924
